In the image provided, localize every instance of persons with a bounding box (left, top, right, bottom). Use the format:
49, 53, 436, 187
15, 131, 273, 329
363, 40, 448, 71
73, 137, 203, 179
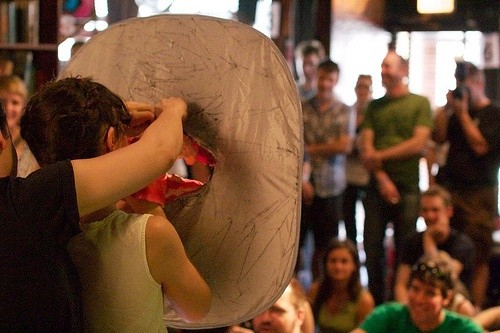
0, 95, 187, 333
226, 39, 500, 333
19, 73, 212, 333
0, 76, 40, 178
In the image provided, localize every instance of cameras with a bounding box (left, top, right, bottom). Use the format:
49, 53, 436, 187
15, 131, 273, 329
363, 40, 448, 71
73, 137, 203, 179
452, 86, 471, 100
